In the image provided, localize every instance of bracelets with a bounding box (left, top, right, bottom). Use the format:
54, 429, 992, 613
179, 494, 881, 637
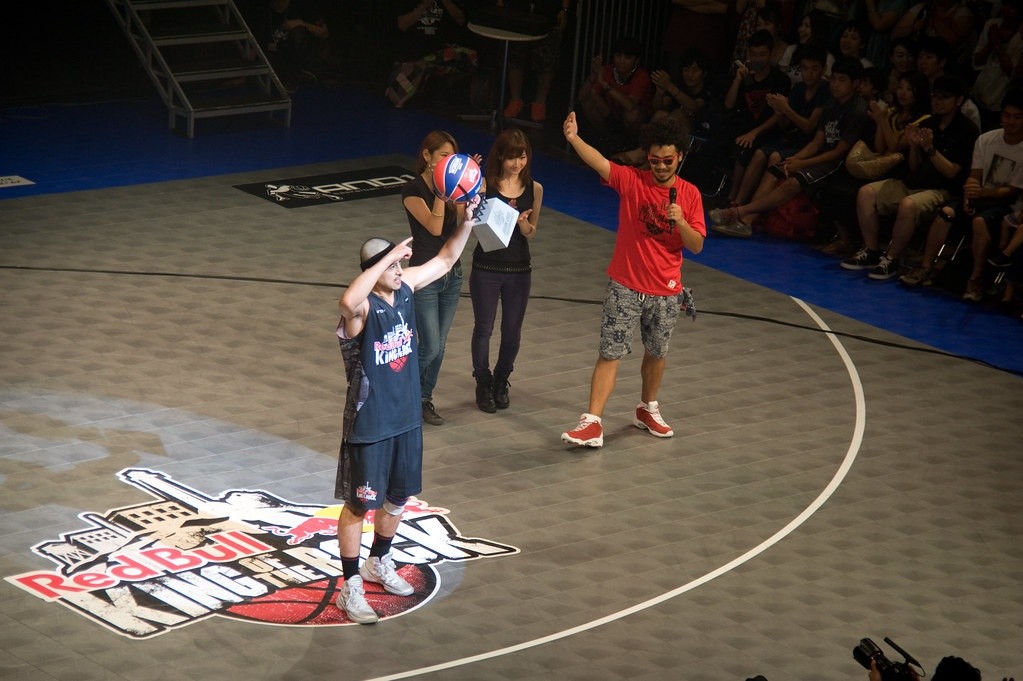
521, 226, 533, 235
432, 211, 444, 218
603, 85, 611, 93
414, 7, 419, 17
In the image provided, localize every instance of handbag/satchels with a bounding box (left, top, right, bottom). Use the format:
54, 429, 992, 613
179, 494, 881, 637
847, 139, 904, 180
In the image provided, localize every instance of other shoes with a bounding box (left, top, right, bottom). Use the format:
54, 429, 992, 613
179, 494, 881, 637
609, 151, 646, 165
709, 207, 737, 225
820, 238, 854, 254
711, 216, 752, 237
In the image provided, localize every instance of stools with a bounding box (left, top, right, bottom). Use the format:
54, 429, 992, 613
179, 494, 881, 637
686, 133, 1007, 284
454, 6, 552, 138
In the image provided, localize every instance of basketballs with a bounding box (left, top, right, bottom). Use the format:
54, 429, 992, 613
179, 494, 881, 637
432, 154, 482, 204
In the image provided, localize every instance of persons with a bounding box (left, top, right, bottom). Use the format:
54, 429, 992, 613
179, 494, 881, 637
578, 0, 1023, 319
336, 202, 485, 624
402, 131, 482, 425
469, 129, 543, 414
560, 109, 707, 449
228, 0, 572, 122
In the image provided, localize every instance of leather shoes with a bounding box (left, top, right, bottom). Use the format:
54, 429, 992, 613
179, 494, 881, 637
476, 375, 496, 412
494, 373, 511, 409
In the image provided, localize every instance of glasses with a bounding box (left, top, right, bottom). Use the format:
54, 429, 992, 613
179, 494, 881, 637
648, 153, 680, 166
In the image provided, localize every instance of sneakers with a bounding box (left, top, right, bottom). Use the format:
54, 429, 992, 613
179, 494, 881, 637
531, 102, 545, 121
1004, 209, 1023, 227
988, 252, 1014, 266
898, 267, 937, 285
840, 247, 882, 268
963, 281, 980, 299
868, 255, 900, 279
633, 404, 674, 437
561, 415, 603, 447
362, 551, 414, 596
504, 100, 523, 118
336, 575, 377, 623
422, 402, 442, 425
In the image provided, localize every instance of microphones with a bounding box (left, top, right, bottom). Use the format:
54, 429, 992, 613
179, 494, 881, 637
884, 636, 922, 668
669, 186, 676, 232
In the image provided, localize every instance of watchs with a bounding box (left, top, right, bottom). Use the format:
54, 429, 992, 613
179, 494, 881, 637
927, 147, 936, 157
561, 7, 568, 12
